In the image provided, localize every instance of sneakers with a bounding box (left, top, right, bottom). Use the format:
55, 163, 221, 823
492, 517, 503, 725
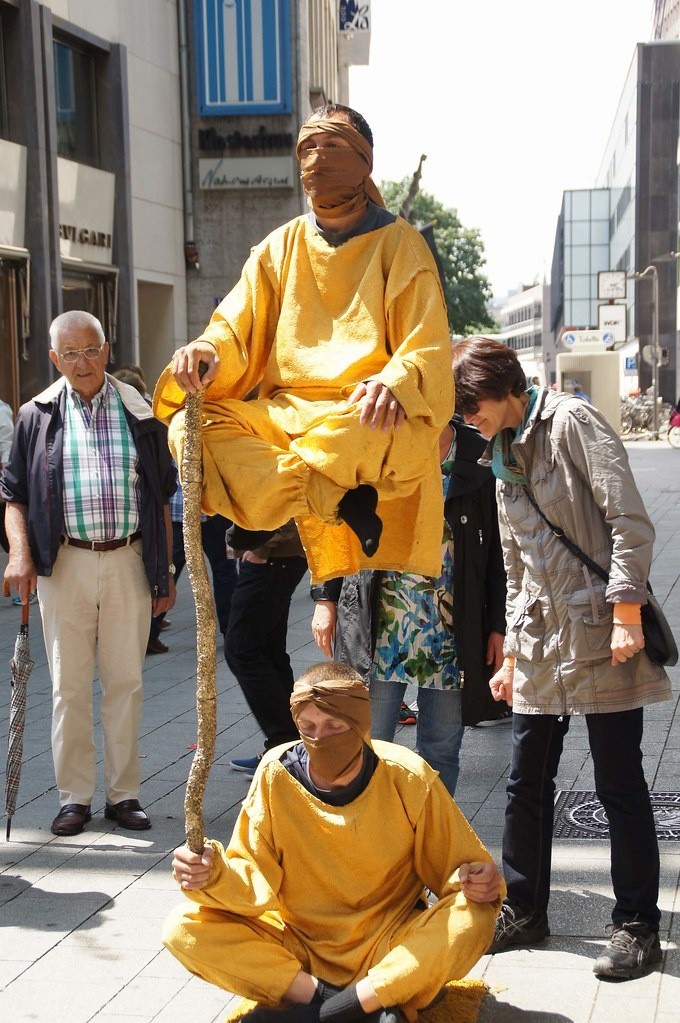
593, 913, 663, 979
396, 702, 417, 723
13, 589, 38, 605
486, 900, 551, 953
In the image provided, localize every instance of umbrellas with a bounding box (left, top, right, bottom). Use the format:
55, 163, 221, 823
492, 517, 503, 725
4, 577, 35, 841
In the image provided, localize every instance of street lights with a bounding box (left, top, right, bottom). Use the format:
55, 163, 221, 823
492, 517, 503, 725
624, 266, 659, 442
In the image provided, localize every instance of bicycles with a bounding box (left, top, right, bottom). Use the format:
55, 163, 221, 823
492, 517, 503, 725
620, 388, 675, 435
667, 425, 680, 449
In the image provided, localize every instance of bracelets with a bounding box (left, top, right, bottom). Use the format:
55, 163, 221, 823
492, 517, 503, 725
315, 598, 329, 601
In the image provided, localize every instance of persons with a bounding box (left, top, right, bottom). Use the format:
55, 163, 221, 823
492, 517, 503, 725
0, 311, 678, 979
152, 104, 456, 587
161, 662, 507, 1023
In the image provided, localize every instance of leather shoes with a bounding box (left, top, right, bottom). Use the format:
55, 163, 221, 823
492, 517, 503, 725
103, 799, 152, 830
52, 802, 92, 835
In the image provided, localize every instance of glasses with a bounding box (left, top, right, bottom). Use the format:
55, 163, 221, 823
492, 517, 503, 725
54, 344, 106, 362
455, 394, 480, 417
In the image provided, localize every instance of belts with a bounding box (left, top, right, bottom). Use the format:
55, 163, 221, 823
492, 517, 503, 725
60, 529, 141, 552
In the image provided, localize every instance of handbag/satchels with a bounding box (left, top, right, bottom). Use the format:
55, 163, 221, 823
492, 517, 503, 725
636, 582, 680, 666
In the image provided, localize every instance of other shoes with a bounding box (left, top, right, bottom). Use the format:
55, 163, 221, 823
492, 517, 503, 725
475, 710, 513, 727
164, 619, 174, 630
242, 768, 255, 778
143, 637, 169, 652
231, 754, 262, 770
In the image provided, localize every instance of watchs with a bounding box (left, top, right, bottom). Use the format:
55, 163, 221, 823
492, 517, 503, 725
166, 561, 176, 574
